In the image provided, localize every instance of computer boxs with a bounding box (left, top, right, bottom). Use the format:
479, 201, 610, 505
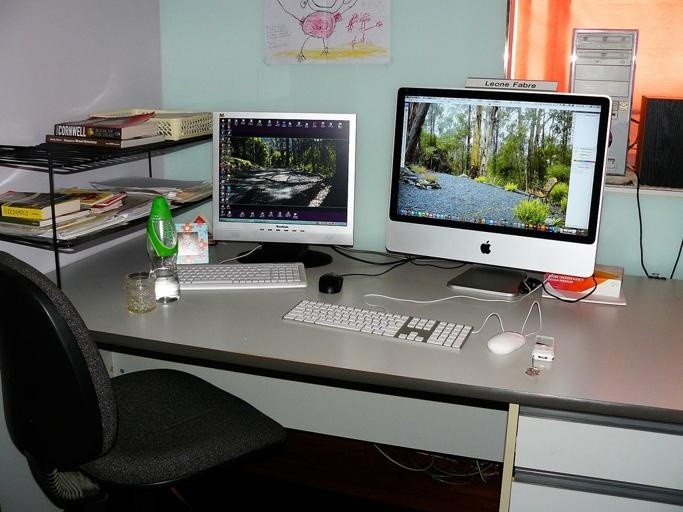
571, 30, 639, 178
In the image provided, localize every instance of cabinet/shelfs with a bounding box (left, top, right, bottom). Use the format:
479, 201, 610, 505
0, 133, 215, 291
499, 403, 683, 512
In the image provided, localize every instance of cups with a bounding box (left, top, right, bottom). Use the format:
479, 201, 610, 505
126, 272, 154, 313
150, 266, 179, 302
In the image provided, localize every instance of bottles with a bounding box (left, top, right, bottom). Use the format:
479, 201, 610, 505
146, 197, 176, 265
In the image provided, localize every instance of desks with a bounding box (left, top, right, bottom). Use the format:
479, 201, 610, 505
43, 232, 683, 463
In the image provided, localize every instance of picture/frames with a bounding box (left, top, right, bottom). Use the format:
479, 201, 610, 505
265, 1, 392, 69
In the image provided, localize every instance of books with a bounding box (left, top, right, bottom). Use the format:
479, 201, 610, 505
54, 110, 157, 139
0, 188, 127, 231
46, 135, 165, 148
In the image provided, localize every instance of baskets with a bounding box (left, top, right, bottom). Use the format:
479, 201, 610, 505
87, 109, 213, 140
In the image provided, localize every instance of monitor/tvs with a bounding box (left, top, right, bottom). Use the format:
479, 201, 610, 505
211, 111, 356, 265
385, 88, 611, 300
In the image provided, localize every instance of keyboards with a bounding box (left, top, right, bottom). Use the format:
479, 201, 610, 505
281, 297, 474, 351
176, 262, 309, 292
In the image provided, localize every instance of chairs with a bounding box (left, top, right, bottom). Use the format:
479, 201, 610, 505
0, 250, 287, 512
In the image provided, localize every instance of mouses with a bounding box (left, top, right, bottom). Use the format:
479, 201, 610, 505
486, 331, 527, 357
319, 272, 344, 294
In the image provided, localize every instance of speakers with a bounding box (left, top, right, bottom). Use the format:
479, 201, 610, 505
636, 95, 683, 188
